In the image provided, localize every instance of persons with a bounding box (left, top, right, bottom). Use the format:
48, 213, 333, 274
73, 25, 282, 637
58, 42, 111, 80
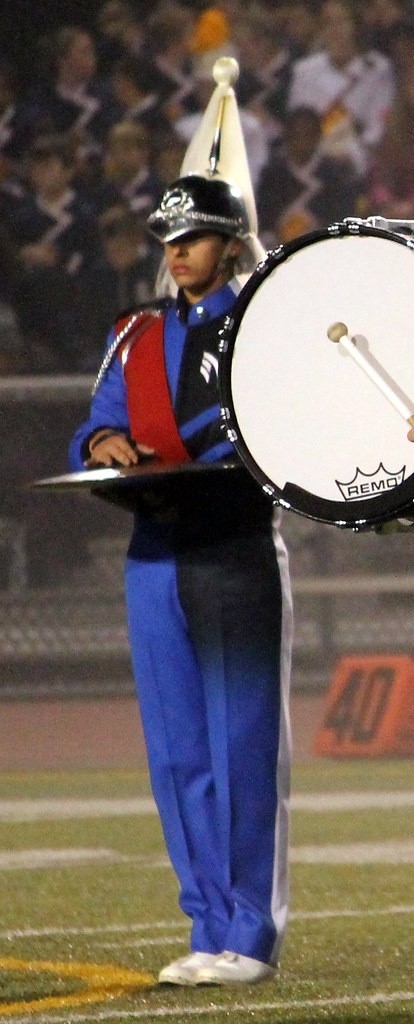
0, 0, 414, 376
66, 176, 293, 985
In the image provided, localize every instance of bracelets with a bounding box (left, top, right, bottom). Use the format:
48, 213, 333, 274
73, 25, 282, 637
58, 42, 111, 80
92, 431, 120, 450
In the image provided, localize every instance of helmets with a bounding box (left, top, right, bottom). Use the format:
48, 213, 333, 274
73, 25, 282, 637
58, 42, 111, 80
146, 176, 258, 277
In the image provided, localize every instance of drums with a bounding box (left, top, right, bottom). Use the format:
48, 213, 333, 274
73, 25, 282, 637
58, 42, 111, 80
212, 212, 414, 535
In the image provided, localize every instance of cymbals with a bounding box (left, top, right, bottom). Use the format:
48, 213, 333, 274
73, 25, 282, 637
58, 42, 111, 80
28, 460, 242, 490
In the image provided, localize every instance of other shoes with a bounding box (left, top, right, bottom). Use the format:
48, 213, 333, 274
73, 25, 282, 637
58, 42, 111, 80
158, 951, 222, 987
196, 949, 276, 987
